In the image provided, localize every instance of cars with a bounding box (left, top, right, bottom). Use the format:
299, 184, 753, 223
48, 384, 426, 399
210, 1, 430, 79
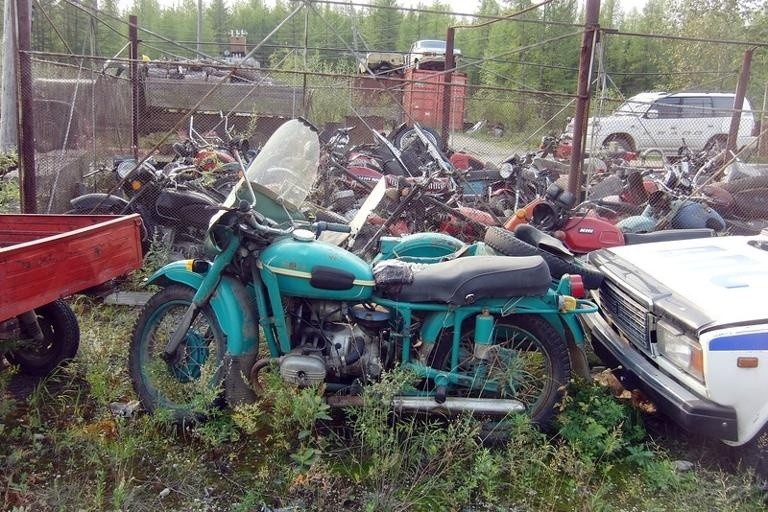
404, 38, 461, 72
577, 225, 767, 468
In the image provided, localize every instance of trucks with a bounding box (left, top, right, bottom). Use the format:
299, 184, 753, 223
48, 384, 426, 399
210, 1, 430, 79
25, 50, 309, 155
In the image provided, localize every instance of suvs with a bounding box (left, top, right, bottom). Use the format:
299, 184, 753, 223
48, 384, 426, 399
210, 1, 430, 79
562, 91, 761, 171
